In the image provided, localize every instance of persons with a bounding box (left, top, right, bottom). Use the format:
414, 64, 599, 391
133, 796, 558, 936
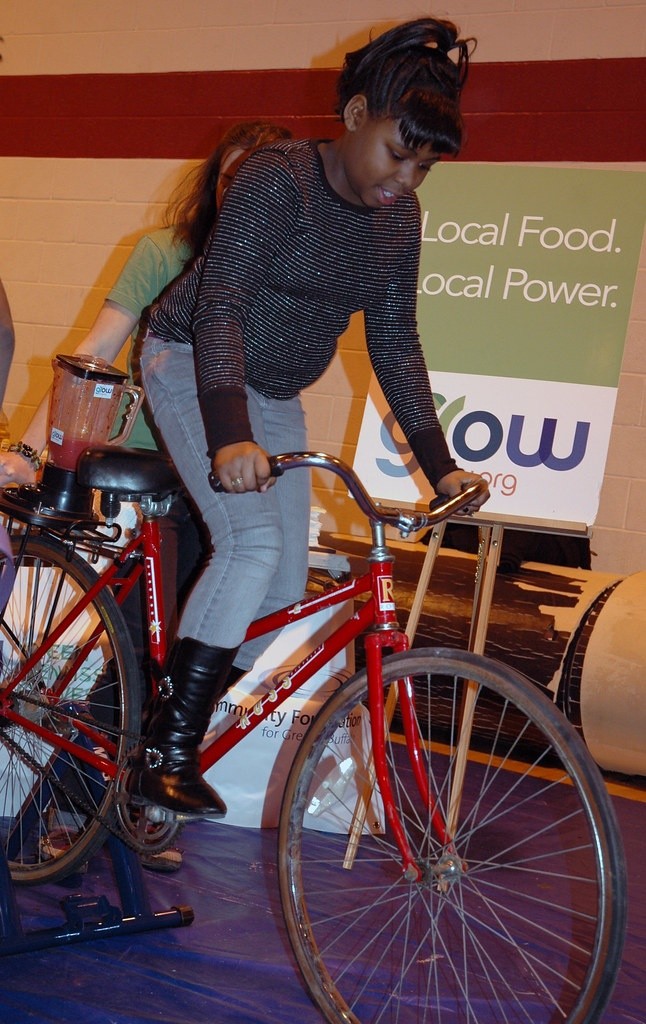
127, 16, 490, 818
0, 278, 16, 442
0, 119, 292, 872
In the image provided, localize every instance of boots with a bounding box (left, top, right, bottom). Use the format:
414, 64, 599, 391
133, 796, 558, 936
131, 638, 247, 825
124, 637, 242, 818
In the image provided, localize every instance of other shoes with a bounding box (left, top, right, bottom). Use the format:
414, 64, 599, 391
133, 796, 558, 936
31, 827, 89, 873
132, 825, 182, 870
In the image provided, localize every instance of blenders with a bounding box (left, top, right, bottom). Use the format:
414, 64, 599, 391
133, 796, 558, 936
19, 354, 145, 520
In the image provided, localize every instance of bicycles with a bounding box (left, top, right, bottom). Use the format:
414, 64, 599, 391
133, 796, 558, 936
0, 451, 625, 1024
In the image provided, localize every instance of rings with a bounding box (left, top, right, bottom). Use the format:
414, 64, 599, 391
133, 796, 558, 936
468, 511, 475, 517
232, 477, 244, 487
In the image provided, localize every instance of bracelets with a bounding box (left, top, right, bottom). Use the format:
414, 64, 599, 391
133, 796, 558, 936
8, 441, 42, 472
